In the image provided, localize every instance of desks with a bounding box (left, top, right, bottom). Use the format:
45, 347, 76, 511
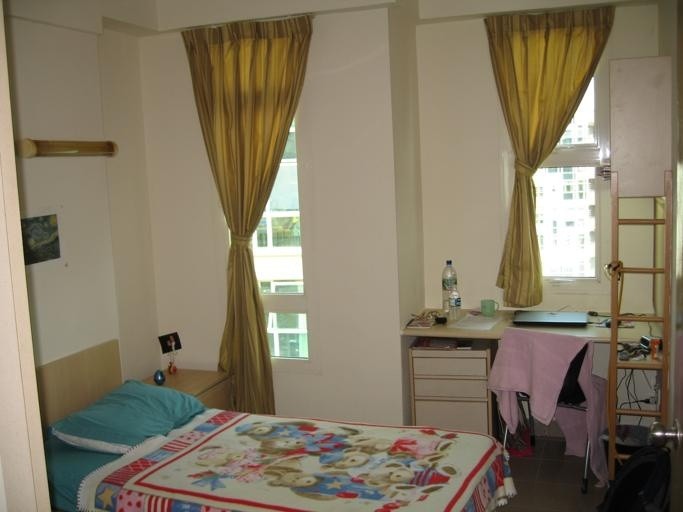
401, 308, 665, 437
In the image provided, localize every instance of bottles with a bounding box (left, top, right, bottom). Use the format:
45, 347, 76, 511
441, 258, 461, 321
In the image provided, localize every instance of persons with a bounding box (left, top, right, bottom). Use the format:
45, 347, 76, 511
27, 223, 58, 264
166, 335, 181, 352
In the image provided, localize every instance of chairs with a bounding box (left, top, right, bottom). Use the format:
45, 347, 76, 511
498, 326, 609, 493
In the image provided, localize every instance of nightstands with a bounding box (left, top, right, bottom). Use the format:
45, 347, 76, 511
140, 366, 232, 410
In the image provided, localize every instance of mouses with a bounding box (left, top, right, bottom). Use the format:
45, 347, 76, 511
606, 318, 621, 328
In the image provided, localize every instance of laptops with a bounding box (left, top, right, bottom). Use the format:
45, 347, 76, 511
513, 310, 588, 325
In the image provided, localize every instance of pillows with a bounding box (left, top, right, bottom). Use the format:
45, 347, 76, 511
51, 378, 207, 455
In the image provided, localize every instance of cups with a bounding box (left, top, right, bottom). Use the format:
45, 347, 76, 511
480, 299, 500, 318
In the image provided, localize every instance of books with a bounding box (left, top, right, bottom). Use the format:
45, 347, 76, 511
407, 319, 432, 329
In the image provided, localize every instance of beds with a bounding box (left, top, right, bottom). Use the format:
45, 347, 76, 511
33, 339, 516, 511
